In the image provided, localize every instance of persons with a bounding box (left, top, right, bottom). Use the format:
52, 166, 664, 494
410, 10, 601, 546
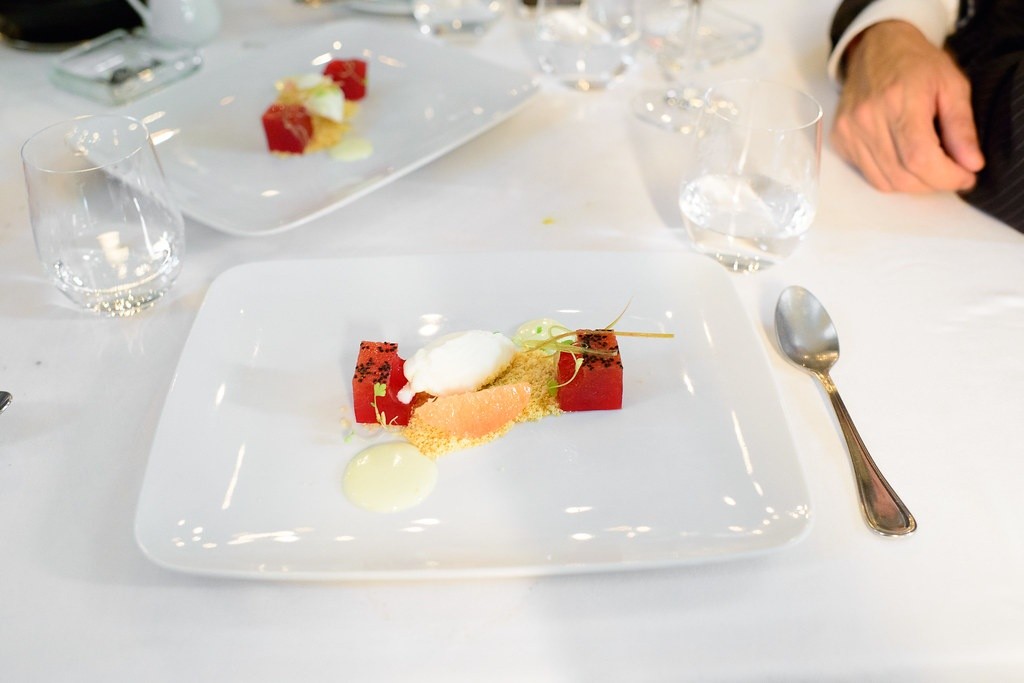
828, 1, 1024, 236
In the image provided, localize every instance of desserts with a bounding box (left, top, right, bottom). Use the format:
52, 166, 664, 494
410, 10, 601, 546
352, 299, 675, 452
261, 59, 367, 153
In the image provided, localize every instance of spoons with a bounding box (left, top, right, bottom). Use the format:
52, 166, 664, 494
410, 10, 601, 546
774, 285, 918, 536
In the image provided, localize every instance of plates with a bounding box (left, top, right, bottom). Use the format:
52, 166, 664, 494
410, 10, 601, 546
69, 19, 543, 236
135, 256, 812, 581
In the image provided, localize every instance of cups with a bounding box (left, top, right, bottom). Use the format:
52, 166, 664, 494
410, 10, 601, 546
676, 76, 825, 274
528, 0, 628, 96
413, 0, 506, 46
130, 0, 220, 48
20, 111, 187, 317
